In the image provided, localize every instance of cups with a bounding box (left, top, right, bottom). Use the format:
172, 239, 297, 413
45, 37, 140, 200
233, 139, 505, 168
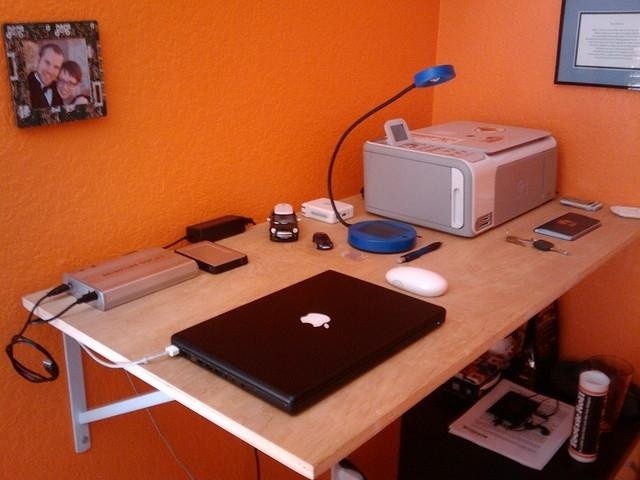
579, 354, 636, 437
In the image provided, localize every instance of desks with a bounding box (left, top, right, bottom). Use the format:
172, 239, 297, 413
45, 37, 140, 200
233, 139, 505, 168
22, 187, 640, 480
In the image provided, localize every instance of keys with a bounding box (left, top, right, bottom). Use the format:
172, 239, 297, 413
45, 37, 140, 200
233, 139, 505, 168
506, 236, 527, 249
533, 238, 570, 256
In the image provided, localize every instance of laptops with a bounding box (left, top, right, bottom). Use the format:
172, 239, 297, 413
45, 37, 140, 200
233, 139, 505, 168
171, 268, 447, 416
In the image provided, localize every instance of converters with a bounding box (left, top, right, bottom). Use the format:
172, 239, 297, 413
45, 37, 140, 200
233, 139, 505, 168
185, 214, 252, 241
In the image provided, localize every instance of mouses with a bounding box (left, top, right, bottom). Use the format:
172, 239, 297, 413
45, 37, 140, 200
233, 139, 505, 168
385, 266, 448, 297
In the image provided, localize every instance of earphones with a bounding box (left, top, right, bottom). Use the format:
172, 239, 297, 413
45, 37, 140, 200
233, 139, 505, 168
535, 424, 550, 436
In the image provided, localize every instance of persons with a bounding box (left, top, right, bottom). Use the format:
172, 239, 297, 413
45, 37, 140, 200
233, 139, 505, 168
53, 59, 91, 106
26, 42, 92, 110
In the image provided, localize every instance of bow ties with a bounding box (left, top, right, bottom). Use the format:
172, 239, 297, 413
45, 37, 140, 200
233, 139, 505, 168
41, 83, 54, 91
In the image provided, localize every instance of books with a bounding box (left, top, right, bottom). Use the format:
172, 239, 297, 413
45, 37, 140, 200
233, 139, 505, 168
534, 209, 604, 241
447, 377, 580, 472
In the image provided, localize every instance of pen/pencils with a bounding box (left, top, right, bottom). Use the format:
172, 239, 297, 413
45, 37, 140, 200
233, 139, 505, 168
397, 242, 443, 264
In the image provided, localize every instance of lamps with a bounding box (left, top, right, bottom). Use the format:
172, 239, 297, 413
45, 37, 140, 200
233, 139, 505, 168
326, 63, 458, 254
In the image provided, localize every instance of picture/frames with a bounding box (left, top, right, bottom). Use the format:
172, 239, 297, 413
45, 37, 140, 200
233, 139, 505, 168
4, 17, 109, 125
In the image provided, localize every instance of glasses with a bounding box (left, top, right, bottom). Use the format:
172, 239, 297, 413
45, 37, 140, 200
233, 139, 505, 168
58, 77, 79, 88
